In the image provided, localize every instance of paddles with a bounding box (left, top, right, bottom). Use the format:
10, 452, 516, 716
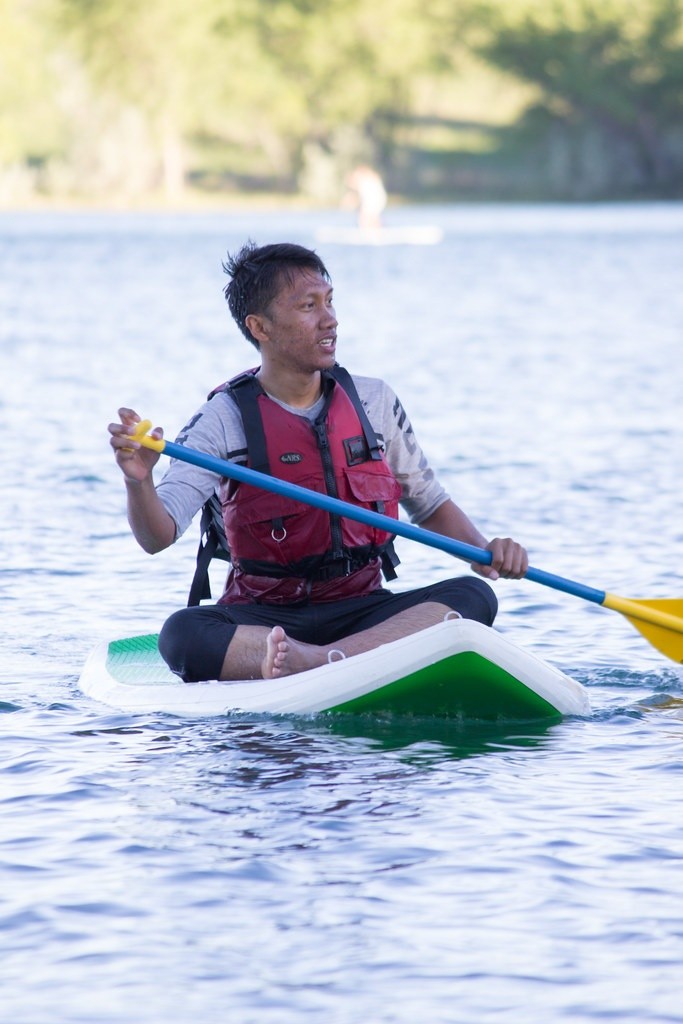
121, 418, 682, 667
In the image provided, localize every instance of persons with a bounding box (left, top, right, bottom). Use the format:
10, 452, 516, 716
108, 242, 529, 681
341, 163, 389, 225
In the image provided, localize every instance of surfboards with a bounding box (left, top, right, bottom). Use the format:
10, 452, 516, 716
76, 619, 589, 721
